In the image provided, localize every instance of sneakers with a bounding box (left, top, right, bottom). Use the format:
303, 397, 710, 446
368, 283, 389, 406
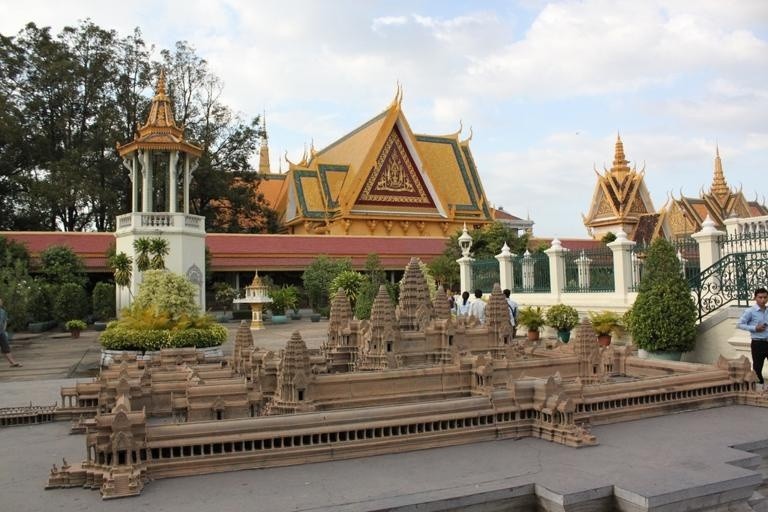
757, 375, 764, 383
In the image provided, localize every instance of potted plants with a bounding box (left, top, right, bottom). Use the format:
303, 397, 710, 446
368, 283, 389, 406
544, 304, 580, 345
513, 304, 547, 342
587, 308, 629, 346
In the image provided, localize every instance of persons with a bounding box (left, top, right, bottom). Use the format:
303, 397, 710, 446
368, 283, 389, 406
447, 295, 457, 312
736, 289, 768, 384
468, 289, 487, 325
504, 289, 519, 343
0, 298, 23, 367
457, 291, 472, 316
